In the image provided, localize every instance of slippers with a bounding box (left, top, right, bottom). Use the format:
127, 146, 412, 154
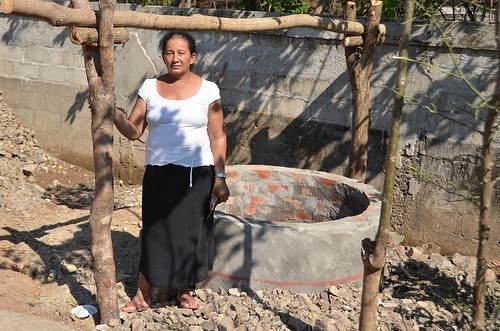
122, 300, 150, 313
176, 290, 199, 309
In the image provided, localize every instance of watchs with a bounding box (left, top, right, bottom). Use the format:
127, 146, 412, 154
215, 173, 226, 178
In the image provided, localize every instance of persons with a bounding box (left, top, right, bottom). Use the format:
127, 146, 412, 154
115, 33, 229, 311
